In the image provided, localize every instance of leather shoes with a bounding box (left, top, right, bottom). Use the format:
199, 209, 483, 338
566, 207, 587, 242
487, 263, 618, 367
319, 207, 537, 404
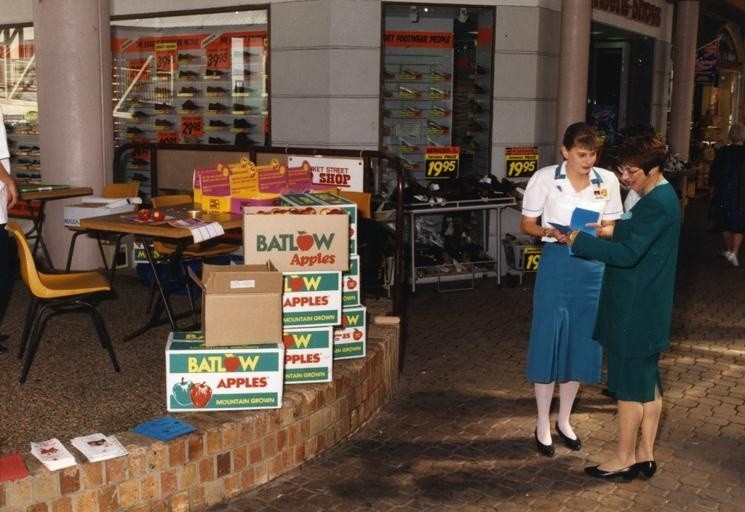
722, 250, 740, 266
555, 420, 582, 451
535, 426, 555, 456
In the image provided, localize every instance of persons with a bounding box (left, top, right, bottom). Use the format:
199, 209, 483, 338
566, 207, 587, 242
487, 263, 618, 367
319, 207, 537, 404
521, 122, 625, 457
0, 105, 19, 353
570, 130, 681, 482
708, 123, 745, 268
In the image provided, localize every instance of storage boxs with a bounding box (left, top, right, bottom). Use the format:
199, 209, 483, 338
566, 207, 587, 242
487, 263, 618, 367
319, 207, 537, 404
165, 194, 368, 414
58, 192, 143, 229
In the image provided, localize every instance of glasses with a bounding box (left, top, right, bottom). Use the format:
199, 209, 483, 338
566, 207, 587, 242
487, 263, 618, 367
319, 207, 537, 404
618, 165, 642, 175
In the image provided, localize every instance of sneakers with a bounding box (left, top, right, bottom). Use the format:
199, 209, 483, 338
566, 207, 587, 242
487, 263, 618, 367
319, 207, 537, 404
15, 144, 41, 181
178, 52, 203, 113
154, 71, 175, 129
126, 97, 147, 136
203, 68, 230, 146
233, 50, 260, 114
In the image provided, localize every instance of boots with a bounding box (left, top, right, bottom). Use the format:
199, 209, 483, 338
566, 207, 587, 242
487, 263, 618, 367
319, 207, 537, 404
233, 119, 261, 145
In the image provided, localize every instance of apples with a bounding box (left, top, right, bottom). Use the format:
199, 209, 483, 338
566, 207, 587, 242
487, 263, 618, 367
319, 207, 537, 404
190, 381, 212, 408
282, 335, 293, 348
289, 278, 304, 291
247, 207, 343, 216
138, 208, 149, 220
347, 277, 357, 289
224, 357, 240, 372
151, 206, 164, 223
296, 234, 314, 251
353, 328, 362, 340
172, 377, 193, 406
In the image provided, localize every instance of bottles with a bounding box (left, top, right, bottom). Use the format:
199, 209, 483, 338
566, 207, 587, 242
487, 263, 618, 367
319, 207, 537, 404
461, 232, 472, 244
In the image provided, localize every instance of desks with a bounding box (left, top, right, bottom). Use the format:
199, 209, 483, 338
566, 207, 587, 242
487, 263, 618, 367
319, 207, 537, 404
5, 184, 94, 277
76, 209, 245, 343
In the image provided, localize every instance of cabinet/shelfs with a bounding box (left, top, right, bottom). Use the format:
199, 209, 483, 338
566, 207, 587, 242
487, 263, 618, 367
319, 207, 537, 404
118, 50, 265, 151
376, 72, 456, 172
403, 195, 518, 293
6, 130, 44, 188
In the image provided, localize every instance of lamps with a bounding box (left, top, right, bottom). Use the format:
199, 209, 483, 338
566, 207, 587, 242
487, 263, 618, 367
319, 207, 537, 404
457, 7, 471, 24
407, 5, 419, 23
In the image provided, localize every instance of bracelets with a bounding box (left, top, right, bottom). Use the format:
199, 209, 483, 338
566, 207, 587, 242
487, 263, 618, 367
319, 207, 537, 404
544, 228, 552, 238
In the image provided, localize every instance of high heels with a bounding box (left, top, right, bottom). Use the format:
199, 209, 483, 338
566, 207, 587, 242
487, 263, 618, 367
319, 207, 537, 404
585, 461, 636, 483
635, 460, 657, 477
397, 66, 418, 147
427, 70, 447, 149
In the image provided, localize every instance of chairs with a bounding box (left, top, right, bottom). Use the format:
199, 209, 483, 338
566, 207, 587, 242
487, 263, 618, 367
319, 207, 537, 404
65, 182, 142, 280
339, 192, 373, 222
1, 219, 121, 386
147, 194, 241, 333
